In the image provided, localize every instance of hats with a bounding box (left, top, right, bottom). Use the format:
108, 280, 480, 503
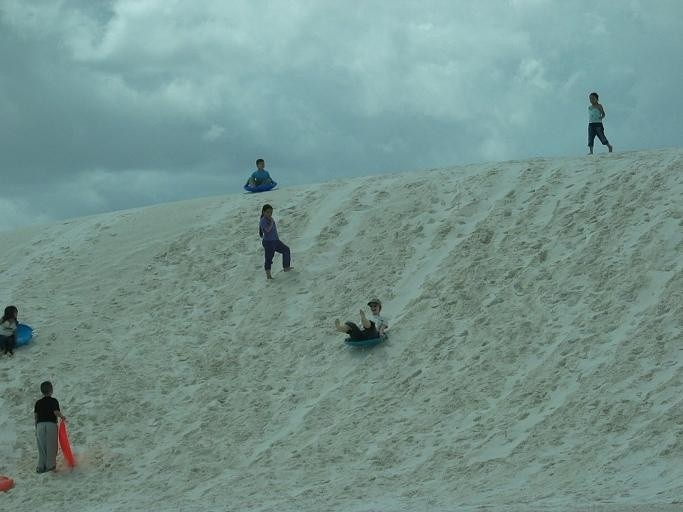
367, 299, 381, 305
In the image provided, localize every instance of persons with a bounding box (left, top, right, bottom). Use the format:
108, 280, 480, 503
258, 203, 295, 280
0, 303, 21, 358
585, 91, 614, 156
244, 157, 274, 189
333, 297, 389, 342
30, 379, 67, 475
